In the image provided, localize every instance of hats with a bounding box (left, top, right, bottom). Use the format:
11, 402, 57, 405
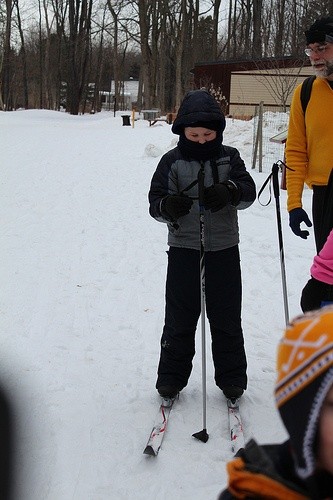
305, 14, 333, 45
274, 307, 333, 481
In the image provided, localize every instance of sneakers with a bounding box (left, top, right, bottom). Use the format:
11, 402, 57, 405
158, 384, 180, 407
223, 385, 244, 408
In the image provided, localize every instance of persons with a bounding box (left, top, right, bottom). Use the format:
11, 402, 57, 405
285, 17, 333, 312
147, 90, 257, 408
219, 303, 333, 500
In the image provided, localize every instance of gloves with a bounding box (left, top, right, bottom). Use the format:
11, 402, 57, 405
203, 179, 241, 214
163, 192, 195, 218
289, 207, 312, 239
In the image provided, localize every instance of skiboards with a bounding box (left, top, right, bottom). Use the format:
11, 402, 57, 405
142, 395, 245, 457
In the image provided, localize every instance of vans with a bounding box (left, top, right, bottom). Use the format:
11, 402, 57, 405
98, 91, 133, 112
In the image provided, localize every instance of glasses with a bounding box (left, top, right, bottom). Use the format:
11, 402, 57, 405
304, 47, 331, 57
